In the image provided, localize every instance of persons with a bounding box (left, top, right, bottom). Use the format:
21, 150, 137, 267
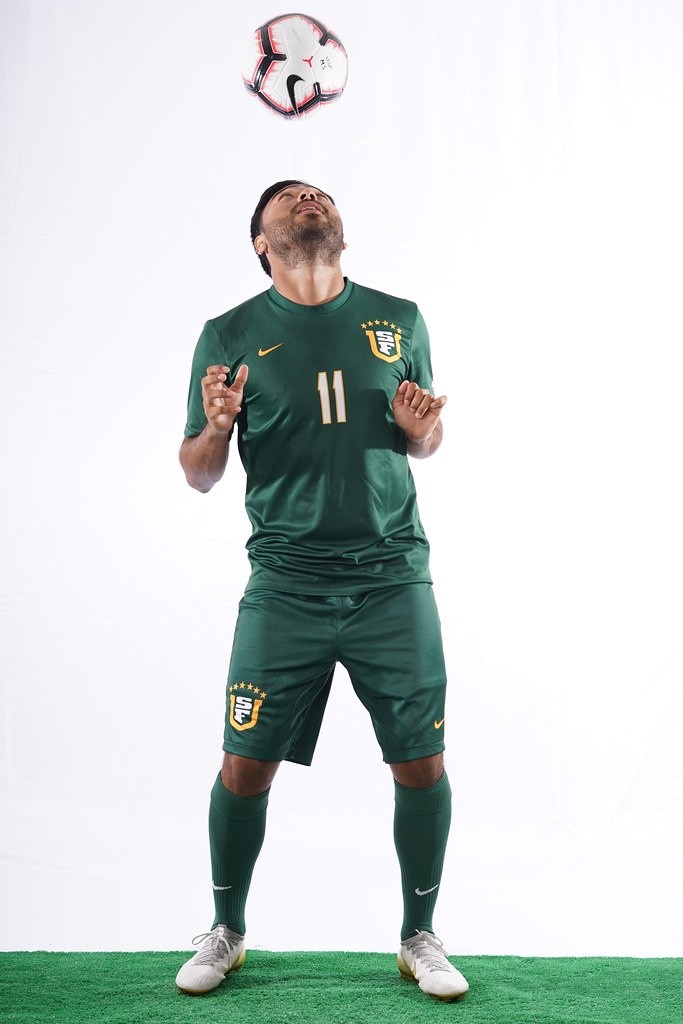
176, 178, 471, 1000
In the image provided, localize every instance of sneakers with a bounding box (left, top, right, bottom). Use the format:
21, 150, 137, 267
395, 927, 470, 1002
175, 924, 247, 994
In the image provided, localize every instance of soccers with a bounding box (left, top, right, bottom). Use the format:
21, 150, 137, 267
242, 13, 348, 127
